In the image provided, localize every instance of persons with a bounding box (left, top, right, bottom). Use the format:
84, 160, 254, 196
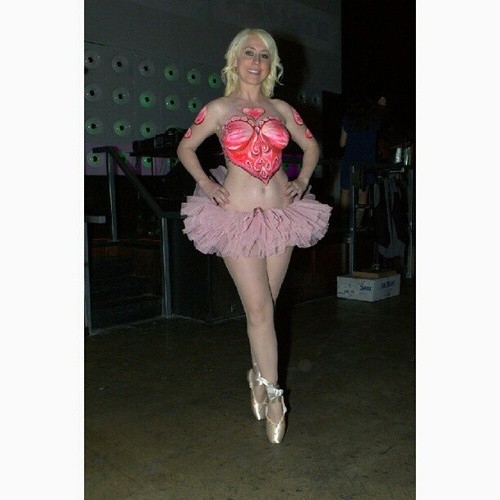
338, 90, 380, 272
176, 28, 334, 445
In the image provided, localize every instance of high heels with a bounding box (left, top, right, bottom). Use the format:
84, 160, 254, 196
265, 393, 287, 444
246, 368, 265, 421
